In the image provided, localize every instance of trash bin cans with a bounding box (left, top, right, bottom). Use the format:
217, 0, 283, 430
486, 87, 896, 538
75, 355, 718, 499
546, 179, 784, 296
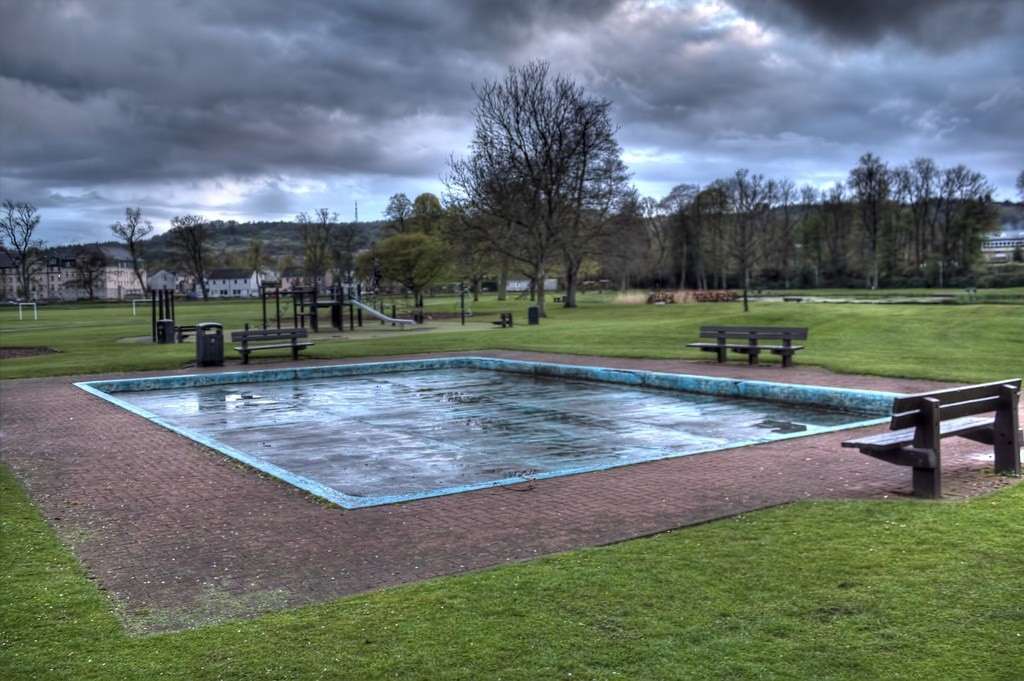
196, 322, 224, 367
157, 319, 174, 344
528, 306, 540, 325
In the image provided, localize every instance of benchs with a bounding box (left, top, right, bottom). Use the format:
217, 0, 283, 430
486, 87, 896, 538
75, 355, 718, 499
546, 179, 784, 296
840, 378, 1024, 499
230, 328, 316, 365
175, 325, 210, 343
687, 325, 807, 368
